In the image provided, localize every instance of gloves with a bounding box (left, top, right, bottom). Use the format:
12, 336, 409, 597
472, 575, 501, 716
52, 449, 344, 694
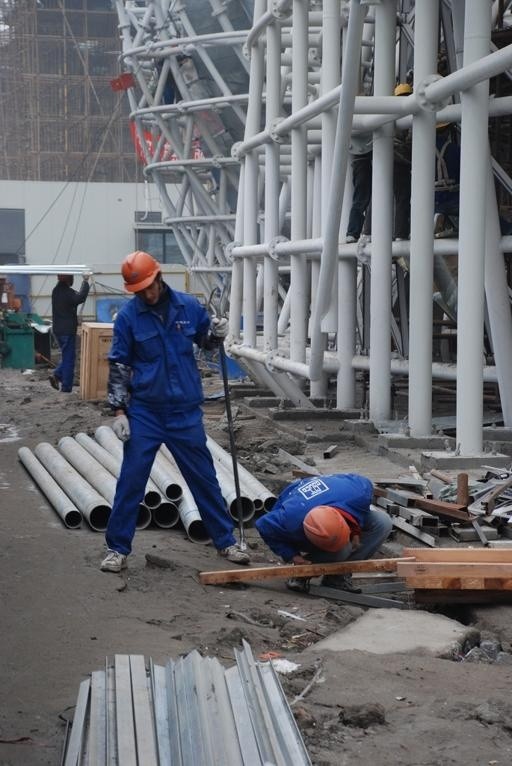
113, 415, 130, 442
210, 318, 228, 338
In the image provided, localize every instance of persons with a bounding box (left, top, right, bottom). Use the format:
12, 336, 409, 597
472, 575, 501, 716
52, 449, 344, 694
154, 44, 193, 106
46, 273, 89, 393
98, 249, 251, 572
343, 83, 460, 244
255, 473, 394, 594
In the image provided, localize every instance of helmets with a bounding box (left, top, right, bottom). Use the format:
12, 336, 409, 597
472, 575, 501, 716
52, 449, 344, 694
121, 252, 160, 292
304, 506, 351, 551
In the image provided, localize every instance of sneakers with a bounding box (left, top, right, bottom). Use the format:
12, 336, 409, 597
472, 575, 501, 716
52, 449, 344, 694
101, 552, 127, 572
49, 374, 59, 391
217, 546, 250, 562
321, 575, 362, 594
287, 576, 306, 592
